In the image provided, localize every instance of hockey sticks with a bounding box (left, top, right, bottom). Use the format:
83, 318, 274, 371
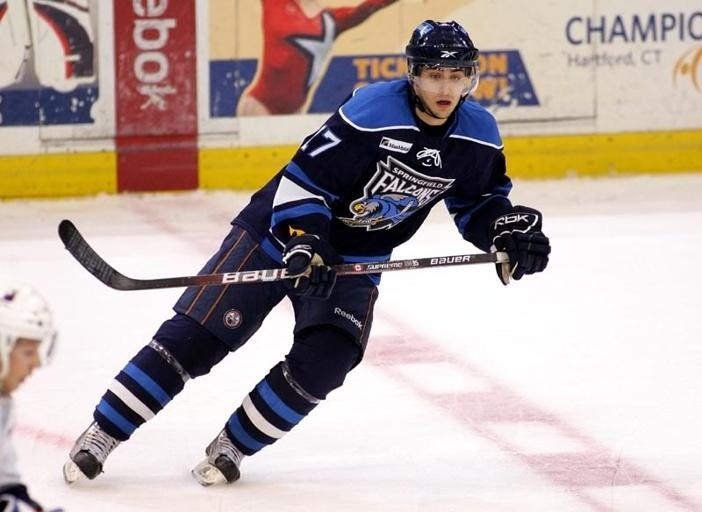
58, 219, 510, 291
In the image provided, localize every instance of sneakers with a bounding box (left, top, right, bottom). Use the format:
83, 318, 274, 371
204, 426, 243, 484
68, 418, 121, 480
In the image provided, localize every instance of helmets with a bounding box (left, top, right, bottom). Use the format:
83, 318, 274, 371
404, 18, 480, 69
0, 289, 57, 393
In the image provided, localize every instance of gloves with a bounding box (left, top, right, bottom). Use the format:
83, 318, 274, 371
280, 231, 340, 303
484, 203, 551, 287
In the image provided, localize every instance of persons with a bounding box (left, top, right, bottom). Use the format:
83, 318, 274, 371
235, 0, 400, 119
68, 18, 551, 486
0, 270, 65, 511
